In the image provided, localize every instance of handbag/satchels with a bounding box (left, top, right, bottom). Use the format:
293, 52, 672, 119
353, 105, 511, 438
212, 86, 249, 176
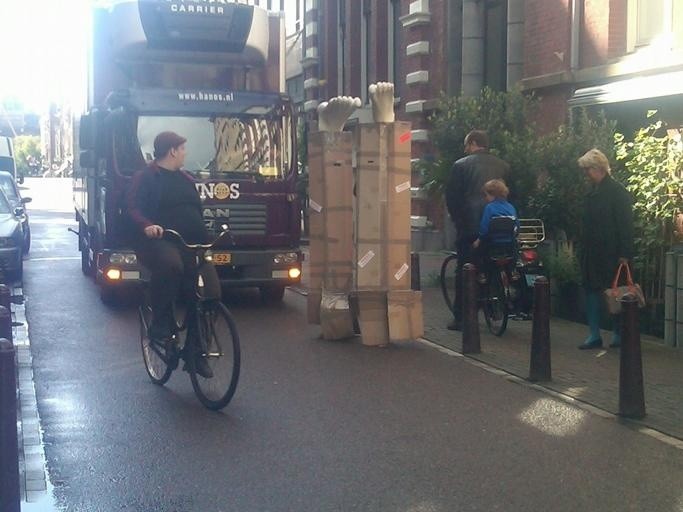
604, 262, 646, 315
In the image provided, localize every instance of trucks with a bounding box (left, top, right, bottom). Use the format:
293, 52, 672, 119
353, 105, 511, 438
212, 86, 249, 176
65, 0, 305, 308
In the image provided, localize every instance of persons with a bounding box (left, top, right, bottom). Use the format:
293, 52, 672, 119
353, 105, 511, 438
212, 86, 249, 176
575, 147, 633, 351
26, 154, 39, 176
316, 94, 362, 133
467, 178, 519, 286
125, 132, 223, 380
367, 81, 396, 124
444, 129, 517, 332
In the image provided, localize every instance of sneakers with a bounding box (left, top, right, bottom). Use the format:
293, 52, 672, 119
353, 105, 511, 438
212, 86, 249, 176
148, 321, 176, 340
476, 275, 488, 284
184, 356, 214, 378
578, 338, 620, 350
448, 322, 464, 330
512, 272, 521, 281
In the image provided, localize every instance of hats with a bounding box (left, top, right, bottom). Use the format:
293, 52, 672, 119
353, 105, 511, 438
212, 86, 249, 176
154, 132, 186, 157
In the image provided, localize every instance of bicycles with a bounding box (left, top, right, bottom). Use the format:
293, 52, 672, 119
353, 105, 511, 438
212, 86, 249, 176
438, 247, 515, 337
134, 223, 243, 415
